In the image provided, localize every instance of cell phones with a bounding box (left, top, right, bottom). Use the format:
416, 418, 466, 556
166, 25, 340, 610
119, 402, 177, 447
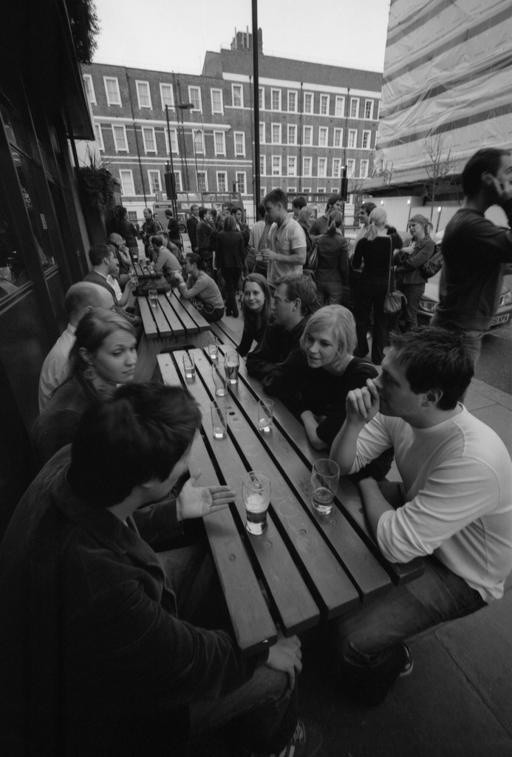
481, 173, 493, 185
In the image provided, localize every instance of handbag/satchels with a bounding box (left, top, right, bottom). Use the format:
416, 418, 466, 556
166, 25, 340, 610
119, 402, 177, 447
132, 274, 170, 295
307, 235, 325, 269
422, 240, 444, 277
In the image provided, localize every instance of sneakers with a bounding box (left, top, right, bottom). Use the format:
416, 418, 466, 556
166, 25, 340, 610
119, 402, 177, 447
251, 720, 323, 757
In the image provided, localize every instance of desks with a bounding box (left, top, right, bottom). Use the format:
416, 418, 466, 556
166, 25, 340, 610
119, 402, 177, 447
134, 262, 163, 278
156, 344, 427, 659
137, 287, 211, 338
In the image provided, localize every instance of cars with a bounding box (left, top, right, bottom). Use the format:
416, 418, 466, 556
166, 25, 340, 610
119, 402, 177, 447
414, 228, 512, 337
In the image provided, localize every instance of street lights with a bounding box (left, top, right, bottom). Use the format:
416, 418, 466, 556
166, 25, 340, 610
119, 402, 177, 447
163, 102, 194, 223
360, 197, 442, 232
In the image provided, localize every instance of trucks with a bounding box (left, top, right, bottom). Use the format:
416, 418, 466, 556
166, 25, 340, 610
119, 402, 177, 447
150, 189, 246, 231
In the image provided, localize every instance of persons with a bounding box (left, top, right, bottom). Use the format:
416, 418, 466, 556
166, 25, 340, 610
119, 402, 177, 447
29, 281, 187, 553
0, 381, 323, 756
86, 188, 436, 365
235, 273, 394, 487
328, 326, 512, 684
430, 148, 512, 405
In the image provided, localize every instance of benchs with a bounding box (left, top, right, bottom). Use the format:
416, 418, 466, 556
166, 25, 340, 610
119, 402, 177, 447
204, 321, 241, 349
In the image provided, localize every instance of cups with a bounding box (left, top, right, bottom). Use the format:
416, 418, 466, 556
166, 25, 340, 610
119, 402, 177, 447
241, 470, 272, 536
181, 352, 198, 378
149, 289, 158, 308
211, 363, 230, 396
308, 457, 340, 516
210, 398, 229, 439
132, 253, 153, 272
223, 351, 240, 384
260, 241, 271, 263
208, 336, 220, 359
257, 398, 274, 434
